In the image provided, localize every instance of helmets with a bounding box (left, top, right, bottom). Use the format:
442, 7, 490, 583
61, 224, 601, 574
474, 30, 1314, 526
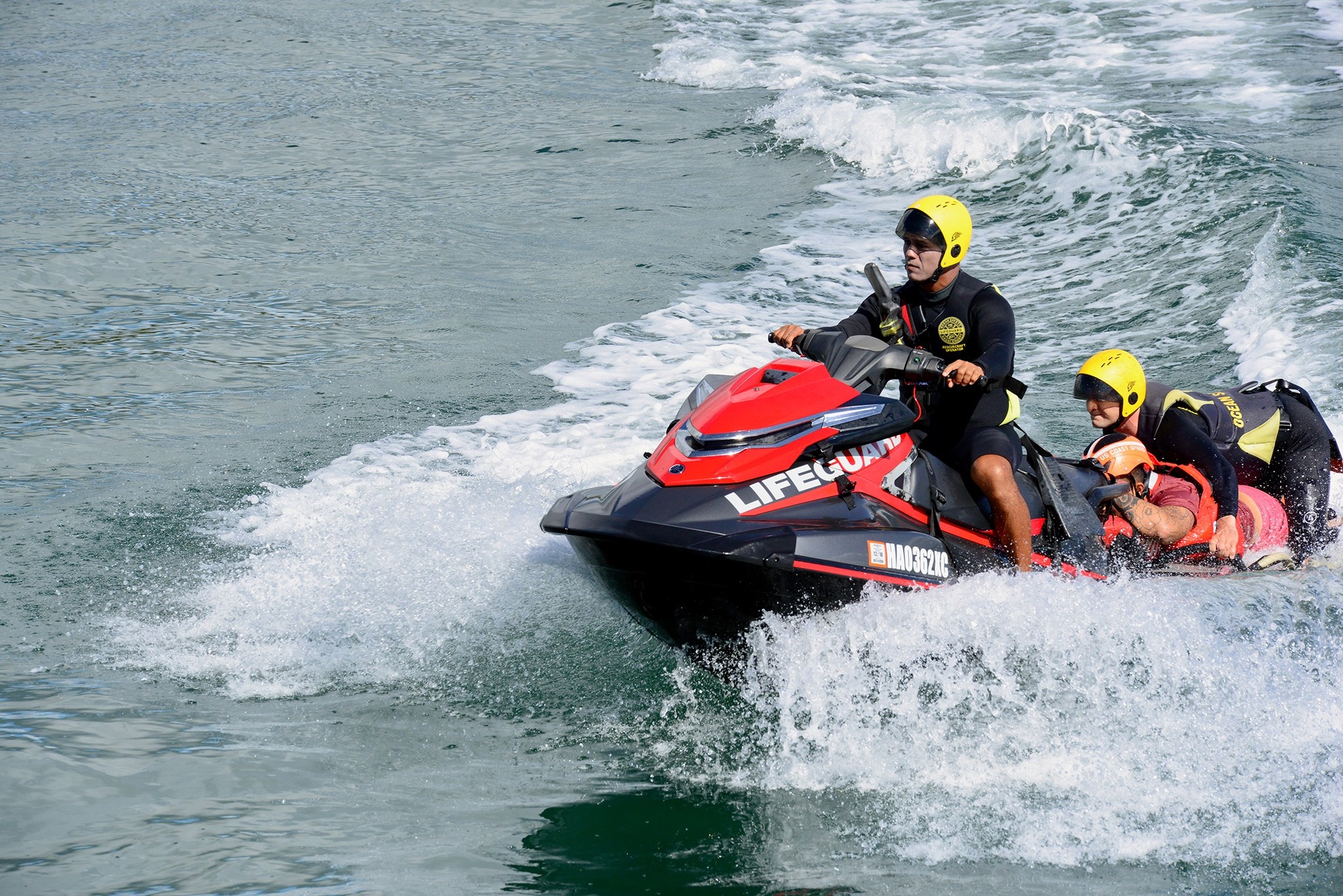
1080, 432, 1155, 480
1073, 349, 1146, 417
895, 194, 972, 269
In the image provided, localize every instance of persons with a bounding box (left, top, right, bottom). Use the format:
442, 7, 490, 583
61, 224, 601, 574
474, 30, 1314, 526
1073, 348, 1343, 571
772, 194, 1034, 575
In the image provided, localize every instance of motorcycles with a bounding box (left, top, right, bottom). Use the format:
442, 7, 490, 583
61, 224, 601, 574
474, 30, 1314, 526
535, 260, 1246, 690
